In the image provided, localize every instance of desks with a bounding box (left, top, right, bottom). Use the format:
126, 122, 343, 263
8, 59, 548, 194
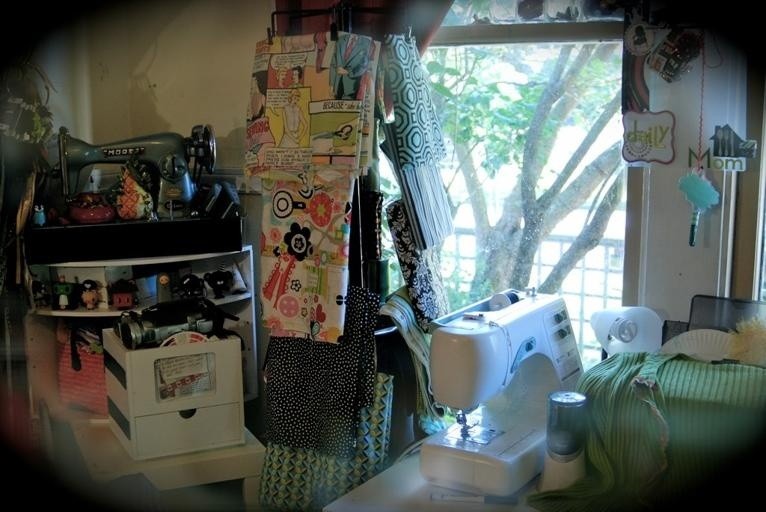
60, 416, 270, 512
320, 447, 541, 511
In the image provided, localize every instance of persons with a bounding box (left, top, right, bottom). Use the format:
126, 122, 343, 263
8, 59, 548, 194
77, 278, 106, 314
30, 198, 46, 230
47, 272, 75, 311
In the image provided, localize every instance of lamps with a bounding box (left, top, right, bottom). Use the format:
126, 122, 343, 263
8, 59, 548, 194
590, 302, 666, 363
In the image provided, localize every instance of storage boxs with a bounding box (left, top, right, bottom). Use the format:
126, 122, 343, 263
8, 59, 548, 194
100, 325, 250, 464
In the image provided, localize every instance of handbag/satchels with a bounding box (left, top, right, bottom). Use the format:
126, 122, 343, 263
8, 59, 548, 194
257, 343, 396, 511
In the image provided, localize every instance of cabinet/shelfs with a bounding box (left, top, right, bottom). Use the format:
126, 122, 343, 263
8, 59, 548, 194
29, 239, 256, 428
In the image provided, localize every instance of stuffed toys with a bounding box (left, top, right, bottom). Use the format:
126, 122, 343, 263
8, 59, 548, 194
203, 267, 234, 300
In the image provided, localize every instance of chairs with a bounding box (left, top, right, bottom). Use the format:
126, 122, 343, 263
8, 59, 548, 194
36, 396, 64, 476
